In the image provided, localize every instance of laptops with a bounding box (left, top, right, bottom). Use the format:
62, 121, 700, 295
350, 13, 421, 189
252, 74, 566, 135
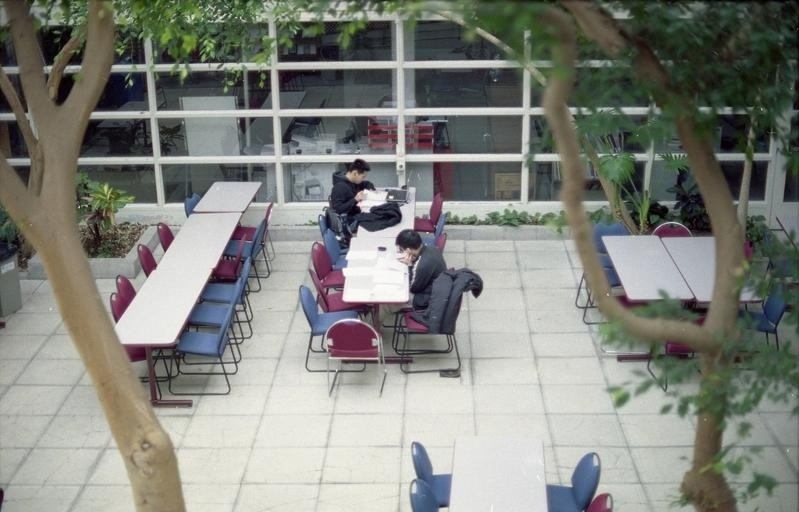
385, 190, 408, 202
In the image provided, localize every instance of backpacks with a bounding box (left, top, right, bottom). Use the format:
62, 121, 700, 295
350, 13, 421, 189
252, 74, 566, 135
323, 207, 349, 249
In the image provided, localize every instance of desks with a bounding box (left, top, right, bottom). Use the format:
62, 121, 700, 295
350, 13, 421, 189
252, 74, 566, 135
243, 89, 309, 149
96, 99, 162, 148
448, 436, 548, 512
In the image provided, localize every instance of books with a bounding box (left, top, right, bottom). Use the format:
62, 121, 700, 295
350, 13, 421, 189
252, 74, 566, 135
357, 185, 388, 210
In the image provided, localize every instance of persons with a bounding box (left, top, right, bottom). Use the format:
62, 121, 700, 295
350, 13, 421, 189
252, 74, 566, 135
330, 159, 374, 220
368, 228, 448, 327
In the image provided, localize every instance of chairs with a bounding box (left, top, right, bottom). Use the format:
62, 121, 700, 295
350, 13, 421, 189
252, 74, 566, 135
409, 480, 440, 512
587, 493, 614, 512
410, 439, 451, 508
573, 217, 787, 392
295, 97, 329, 141
546, 452, 601, 512
